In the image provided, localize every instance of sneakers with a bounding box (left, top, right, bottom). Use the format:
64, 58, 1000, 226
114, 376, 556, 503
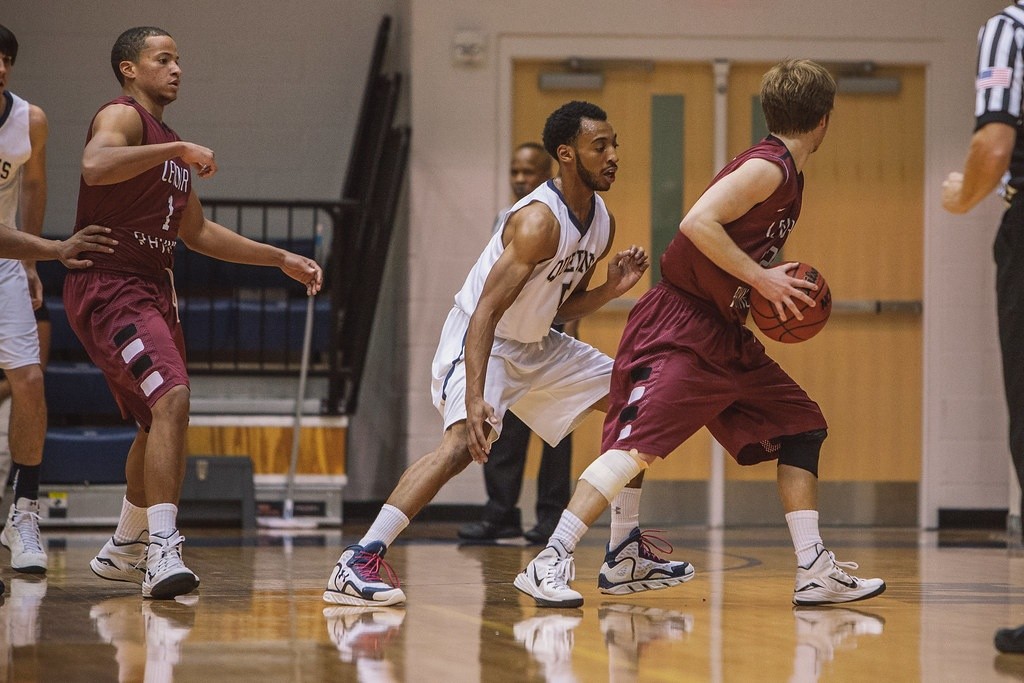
597, 526, 695, 596
142, 529, 196, 599
0, 579, 47, 646
0, 497, 48, 574
513, 539, 584, 608
792, 549, 886, 606
323, 607, 407, 664
514, 609, 583, 663
89, 593, 145, 644
323, 540, 406, 606
525, 524, 556, 543
89, 530, 200, 589
141, 596, 199, 664
794, 606, 885, 659
598, 603, 694, 659
457, 521, 523, 539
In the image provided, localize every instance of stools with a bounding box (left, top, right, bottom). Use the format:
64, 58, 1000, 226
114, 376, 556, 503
177, 456, 255, 529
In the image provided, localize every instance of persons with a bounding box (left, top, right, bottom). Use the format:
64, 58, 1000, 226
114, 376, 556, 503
942, 1, 1024, 656
324, 58, 886, 605
0, 26, 322, 600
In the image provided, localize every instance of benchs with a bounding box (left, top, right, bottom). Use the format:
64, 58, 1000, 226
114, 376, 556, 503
0, 237, 334, 484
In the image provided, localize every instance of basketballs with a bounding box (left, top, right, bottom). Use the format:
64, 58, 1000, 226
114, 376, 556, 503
750, 263, 832, 343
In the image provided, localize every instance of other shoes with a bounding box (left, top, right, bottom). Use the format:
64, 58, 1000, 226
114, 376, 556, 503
0, 580, 4, 594
994, 624, 1024, 654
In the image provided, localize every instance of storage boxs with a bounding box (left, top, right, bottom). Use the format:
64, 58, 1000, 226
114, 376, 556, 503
253, 474, 349, 529
